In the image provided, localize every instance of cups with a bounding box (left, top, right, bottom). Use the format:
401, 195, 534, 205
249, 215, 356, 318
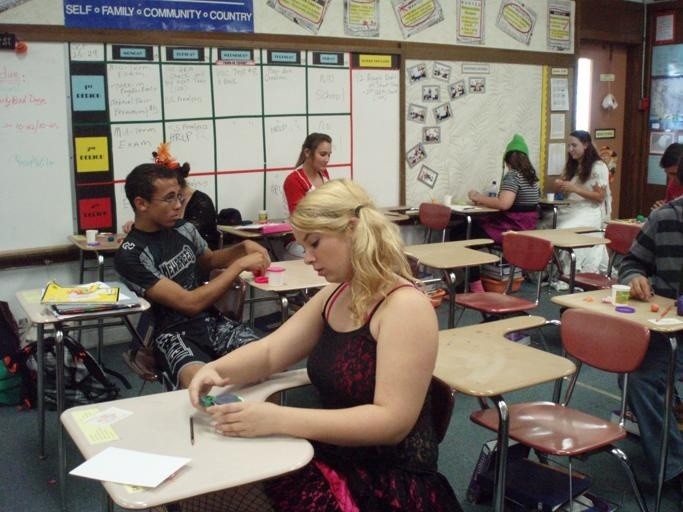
266, 266, 285, 288
611, 284, 631, 307
444, 194, 452, 204
86, 230, 100, 246
547, 194, 555, 203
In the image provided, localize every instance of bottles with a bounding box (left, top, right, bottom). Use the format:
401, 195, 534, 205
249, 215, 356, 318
258, 209, 268, 224
488, 180, 499, 198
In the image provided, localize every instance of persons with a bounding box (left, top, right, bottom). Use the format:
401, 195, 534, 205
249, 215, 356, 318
543, 131, 616, 293
111, 164, 271, 387
190, 178, 461, 510
617, 156, 683, 506
121, 154, 218, 251
282, 133, 332, 258
455, 135, 540, 294
651, 143, 683, 210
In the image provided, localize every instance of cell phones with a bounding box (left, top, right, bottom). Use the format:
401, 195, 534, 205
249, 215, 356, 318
200, 393, 245, 408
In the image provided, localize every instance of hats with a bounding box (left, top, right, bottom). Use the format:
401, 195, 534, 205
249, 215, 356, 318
504, 134, 529, 158
217, 208, 253, 225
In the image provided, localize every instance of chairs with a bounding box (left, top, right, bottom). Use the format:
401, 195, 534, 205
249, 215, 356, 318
560, 223, 641, 291
409, 204, 451, 275
444, 231, 553, 349
472, 311, 650, 512
217, 230, 224, 249
421, 375, 457, 441
120, 269, 246, 396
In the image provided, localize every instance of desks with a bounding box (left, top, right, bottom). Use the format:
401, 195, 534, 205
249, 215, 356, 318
606, 218, 648, 227
73, 233, 126, 366
550, 285, 683, 512
217, 219, 289, 259
446, 203, 500, 294
240, 260, 326, 325
451, 205, 503, 294
433, 316, 577, 512
540, 199, 576, 229
382, 204, 408, 222
18, 282, 144, 507
502, 226, 608, 295
404, 239, 499, 329
61, 369, 311, 512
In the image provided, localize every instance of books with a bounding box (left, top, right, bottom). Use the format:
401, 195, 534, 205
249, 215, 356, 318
39, 280, 141, 314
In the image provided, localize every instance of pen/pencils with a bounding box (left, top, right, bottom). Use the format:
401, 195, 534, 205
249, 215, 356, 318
190, 418, 194, 445
657, 306, 672, 321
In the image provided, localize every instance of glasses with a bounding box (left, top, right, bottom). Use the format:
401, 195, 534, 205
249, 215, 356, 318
151, 192, 183, 203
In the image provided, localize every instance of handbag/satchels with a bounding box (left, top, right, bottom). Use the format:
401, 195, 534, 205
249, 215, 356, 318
0, 359, 37, 406
16, 318, 74, 350
0, 301, 22, 374
21, 337, 120, 411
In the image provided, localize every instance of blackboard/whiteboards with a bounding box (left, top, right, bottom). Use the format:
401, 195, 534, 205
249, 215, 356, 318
0, 23, 405, 269
